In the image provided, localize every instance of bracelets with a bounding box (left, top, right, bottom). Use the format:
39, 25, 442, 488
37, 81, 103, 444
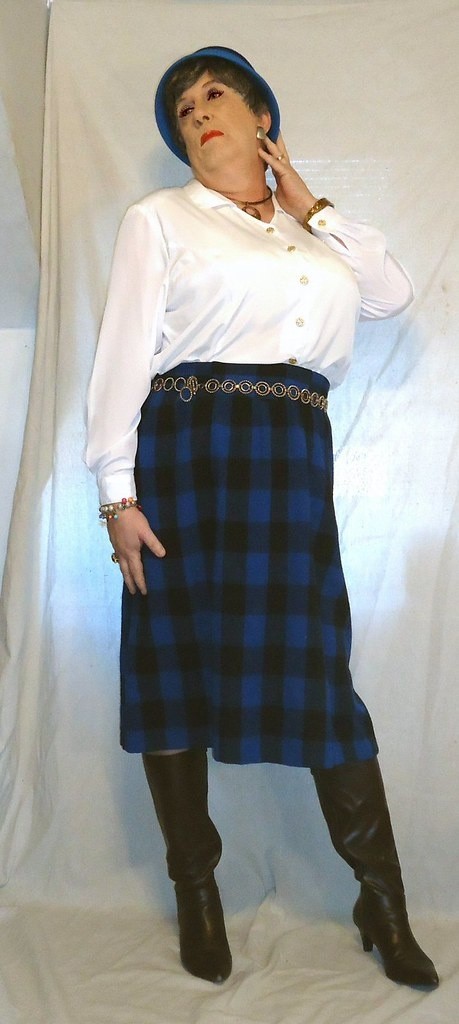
302, 198, 335, 232
99, 497, 143, 520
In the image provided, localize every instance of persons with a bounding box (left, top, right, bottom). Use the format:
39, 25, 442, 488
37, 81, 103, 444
83, 46, 441, 993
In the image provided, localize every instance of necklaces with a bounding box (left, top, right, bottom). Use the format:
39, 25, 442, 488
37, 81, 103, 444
229, 184, 273, 220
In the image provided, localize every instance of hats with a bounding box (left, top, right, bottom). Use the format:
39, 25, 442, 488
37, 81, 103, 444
154, 46, 280, 167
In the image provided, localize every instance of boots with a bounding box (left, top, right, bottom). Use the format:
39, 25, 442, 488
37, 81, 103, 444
311, 755, 440, 986
142, 747, 232, 985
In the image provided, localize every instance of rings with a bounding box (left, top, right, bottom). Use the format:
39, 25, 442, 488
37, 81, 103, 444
277, 154, 285, 161
111, 553, 119, 562
